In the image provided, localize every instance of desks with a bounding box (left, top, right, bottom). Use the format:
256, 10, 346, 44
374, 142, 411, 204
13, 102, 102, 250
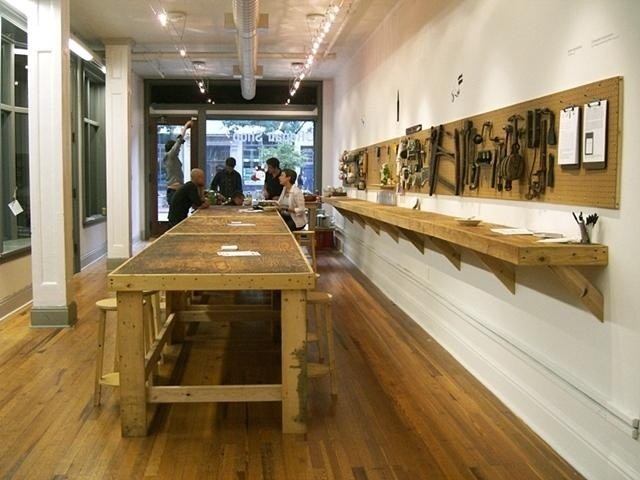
105, 203, 315, 438
303, 201, 321, 230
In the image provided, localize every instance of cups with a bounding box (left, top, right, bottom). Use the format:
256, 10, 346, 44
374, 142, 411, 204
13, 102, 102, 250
579, 223, 593, 244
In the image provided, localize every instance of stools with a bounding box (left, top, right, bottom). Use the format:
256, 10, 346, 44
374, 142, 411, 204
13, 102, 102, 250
94, 291, 166, 408
292, 230, 342, 414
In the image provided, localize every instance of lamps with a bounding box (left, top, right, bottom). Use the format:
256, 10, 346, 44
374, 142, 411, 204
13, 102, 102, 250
68, 5, 340, 106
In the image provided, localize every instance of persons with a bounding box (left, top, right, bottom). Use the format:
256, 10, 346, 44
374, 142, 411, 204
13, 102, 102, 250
167, 167, 212, 231
275, 168, 307, 233
297, 170, 307, 189
224, 191, 246, 206
210, 157, 243, 203
161, 119, 195, 206
261, 158, 284, 201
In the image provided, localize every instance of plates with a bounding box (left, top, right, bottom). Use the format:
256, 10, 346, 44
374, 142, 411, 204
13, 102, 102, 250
258, 202, 275, 206
456, 220, 481, 227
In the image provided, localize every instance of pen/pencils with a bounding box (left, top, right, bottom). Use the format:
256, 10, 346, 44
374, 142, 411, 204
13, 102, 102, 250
572, 211, 599, 244
